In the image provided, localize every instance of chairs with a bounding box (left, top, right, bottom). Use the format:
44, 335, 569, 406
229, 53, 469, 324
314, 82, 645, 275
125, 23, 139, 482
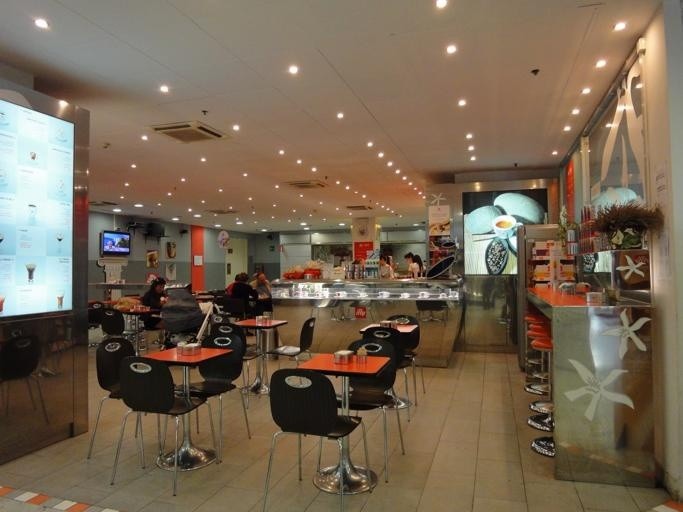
413, 300, 449, 325
317, 340, 405, 486
308, 300, 339, 322
385, 314, 426, 394
362, 326, 413, 424
110, 356, 220, 496
175, 331, 253, 452
88, 300, 105, 349
216, 296, 246, 322
0, 334, 53, 427
265, 316, 318, 385
86, 338, 169, 459
210, 322, 263, 409
101, 307, 137, 341
348, 300, 377, 322
261, 366, 374, 512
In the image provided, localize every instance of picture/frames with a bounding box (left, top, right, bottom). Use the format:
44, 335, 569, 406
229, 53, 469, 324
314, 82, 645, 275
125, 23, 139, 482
589, 199, 663, 252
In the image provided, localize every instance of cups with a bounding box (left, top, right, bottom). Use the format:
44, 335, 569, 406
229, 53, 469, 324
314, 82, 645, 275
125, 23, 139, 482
26, 264, 36, 281
56, 289, 64, 308
493, 215, 524, 240
57, 233, 64, 241
30, 152, 38, 160
0, 295, 5, 313
0, 234, 4, 243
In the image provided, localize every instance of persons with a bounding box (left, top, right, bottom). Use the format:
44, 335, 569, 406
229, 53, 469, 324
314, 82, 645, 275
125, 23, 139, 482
231, 272, 258, 337
379, 254, 395, 280
409, 255, 427, 277
104, 241, 115, 251
404, 252, 414, 263
224, 274, 239, 298
141, 277, 170, 330
248, 271, 273, 316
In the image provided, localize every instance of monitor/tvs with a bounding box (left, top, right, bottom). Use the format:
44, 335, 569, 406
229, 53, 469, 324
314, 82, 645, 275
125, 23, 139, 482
0, 94, 76, 319
100, 229, 131, 255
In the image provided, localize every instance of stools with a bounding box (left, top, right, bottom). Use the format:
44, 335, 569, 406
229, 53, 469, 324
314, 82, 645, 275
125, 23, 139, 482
528, 322, 552, 415
525, 329, 555, 434
524, 312, 548, 395
529, 336, 555, 457
